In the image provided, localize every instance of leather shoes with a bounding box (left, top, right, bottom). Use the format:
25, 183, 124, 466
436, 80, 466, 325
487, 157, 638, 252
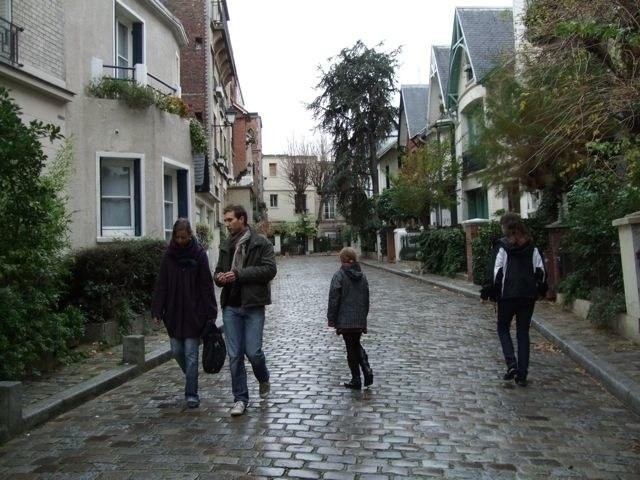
188, 401, 199, 407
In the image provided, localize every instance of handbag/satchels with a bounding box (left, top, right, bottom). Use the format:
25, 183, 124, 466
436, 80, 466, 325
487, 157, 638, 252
202, 322, 226, 374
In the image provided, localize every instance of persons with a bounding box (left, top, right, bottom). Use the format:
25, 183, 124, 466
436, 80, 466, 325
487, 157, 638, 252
481, 213, 548, 388
214, 205, 277, 416
151, 217, 217, 409
327, 246, 373, 390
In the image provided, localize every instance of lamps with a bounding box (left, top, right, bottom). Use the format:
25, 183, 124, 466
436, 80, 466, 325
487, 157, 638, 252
212, 111, 236, 127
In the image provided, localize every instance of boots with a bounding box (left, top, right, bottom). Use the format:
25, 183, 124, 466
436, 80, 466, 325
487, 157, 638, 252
344, 348, 373, 388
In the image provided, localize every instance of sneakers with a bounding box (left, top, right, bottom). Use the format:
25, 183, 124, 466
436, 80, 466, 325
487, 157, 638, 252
504, 364, 526, 386
259, 380, 269, 399
230, 401, 246, 416
